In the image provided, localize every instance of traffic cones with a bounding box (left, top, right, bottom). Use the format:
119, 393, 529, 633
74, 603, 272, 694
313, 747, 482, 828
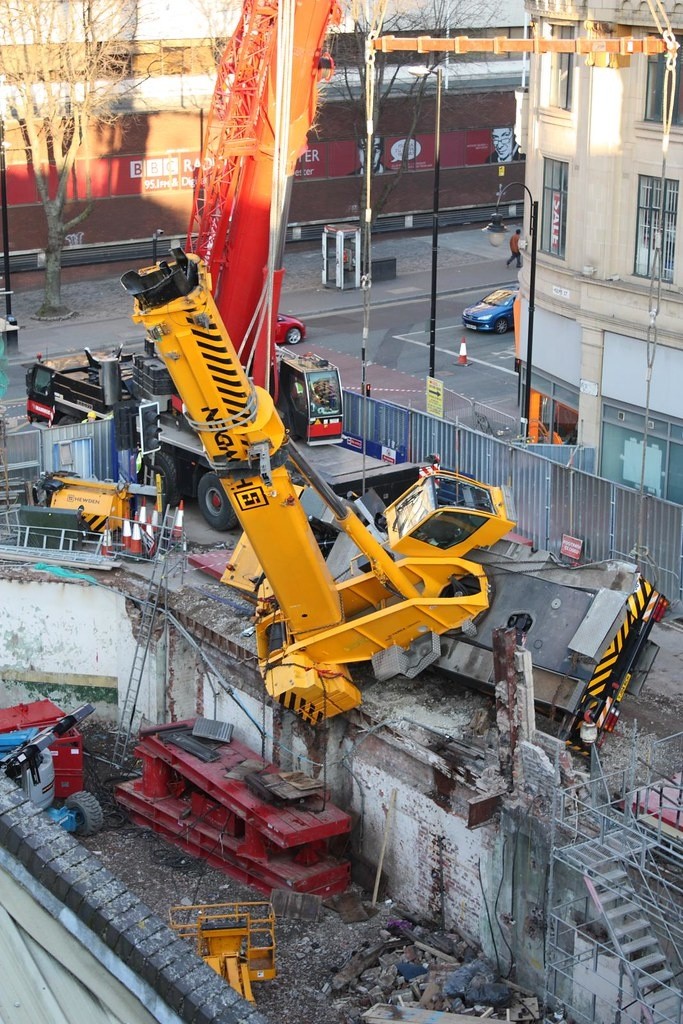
168, 498, 183, 545
148, 502, 161, 534
452, 335, 474, 367
102, 517, 116, 557
144, 513, 158, 559
128, 514, 142, 557
139, 496, 148, 531
121, 510, 132, 553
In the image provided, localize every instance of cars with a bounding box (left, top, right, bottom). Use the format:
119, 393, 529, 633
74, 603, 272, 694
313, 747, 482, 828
275, 312, 306, 346
461, 286, 520, 335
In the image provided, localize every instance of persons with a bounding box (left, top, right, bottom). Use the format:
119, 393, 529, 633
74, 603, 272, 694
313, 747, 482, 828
82, 412, 96, 424
506, 229, 522, 268
344, 248, 348, 265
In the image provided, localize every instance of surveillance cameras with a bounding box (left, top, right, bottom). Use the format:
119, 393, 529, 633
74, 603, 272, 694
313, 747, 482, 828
158, 230, 164, 234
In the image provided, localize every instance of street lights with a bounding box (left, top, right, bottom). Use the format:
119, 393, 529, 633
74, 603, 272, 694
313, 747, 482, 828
481, 181, 539, 444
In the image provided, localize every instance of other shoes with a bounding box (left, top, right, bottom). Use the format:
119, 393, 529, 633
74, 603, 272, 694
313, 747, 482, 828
507, 263, 509, 267
516, 265, 523, 268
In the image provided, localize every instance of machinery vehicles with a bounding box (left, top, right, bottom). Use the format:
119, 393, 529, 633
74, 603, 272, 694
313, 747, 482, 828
23, 1, 444, 523
117, 227, 673, 765
